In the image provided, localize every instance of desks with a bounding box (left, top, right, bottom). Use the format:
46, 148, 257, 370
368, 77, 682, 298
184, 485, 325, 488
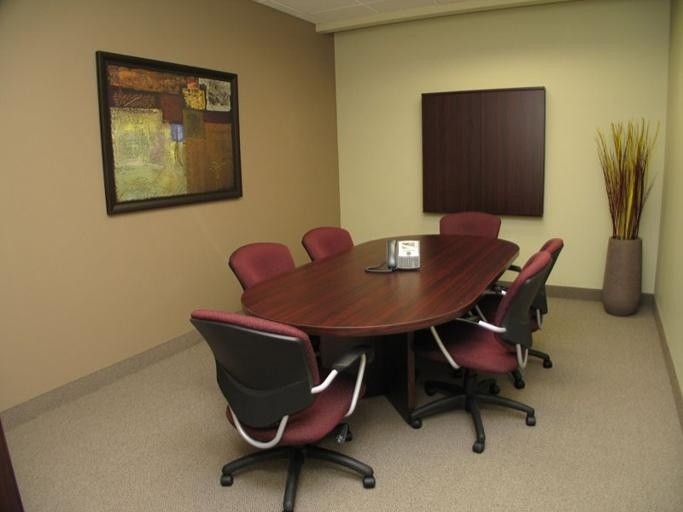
241, 234, 521, 411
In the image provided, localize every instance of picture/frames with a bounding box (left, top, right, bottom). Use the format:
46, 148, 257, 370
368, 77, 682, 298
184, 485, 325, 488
95, 47, 244, 213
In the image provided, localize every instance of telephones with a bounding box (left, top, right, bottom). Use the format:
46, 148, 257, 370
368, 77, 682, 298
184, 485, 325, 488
388, 239, 420, 270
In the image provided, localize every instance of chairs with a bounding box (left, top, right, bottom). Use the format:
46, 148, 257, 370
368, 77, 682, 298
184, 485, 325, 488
300, 227, 354, 262
438, 211, 505, 237
408, 238, 567, 453
188, 308, 377, 500
227, 243, 294, 295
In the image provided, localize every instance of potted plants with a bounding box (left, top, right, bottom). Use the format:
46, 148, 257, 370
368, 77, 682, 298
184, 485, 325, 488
591, 115, 663, 315
